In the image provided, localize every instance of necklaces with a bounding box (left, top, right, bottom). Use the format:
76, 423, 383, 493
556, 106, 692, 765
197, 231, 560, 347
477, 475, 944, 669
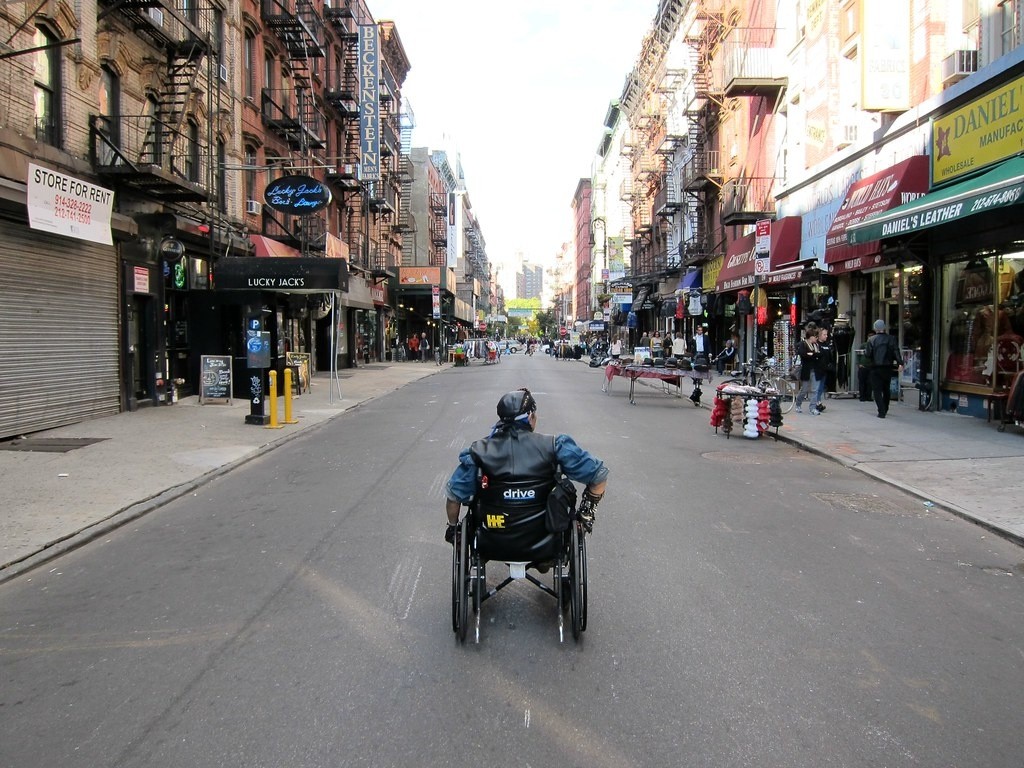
804, 339, 814, 351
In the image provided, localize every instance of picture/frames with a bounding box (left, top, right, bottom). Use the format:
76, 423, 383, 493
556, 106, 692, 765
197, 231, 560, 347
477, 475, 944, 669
902, 350, 914, 383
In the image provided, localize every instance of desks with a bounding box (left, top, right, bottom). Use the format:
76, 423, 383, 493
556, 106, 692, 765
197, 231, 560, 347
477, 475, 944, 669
715, 389, 783, 442
602, 365, 685, 398
448, 350, 456, 363
287, 365, 301, 394
621, 366, 714, 408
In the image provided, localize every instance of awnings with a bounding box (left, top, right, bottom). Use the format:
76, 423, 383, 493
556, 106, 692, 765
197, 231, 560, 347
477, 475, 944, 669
244, 232, 376, 311
608, 215, 811, 312
844, 153, 1024, 248
824, 155, 930, 276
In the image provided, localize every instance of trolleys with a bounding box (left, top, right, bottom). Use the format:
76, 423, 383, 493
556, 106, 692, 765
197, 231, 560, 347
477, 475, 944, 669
997, 372, 1024, 433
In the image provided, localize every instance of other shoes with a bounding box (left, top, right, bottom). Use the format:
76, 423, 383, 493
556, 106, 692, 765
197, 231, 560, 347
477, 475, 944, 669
817, 404, 826, 411
810, 408, 820, 415
795, 405, 802, 413
878, 413, 886, 418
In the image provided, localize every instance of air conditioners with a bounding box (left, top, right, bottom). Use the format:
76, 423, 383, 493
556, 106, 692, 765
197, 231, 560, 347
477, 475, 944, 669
144, 7, 163, 27
246, 200, 261, 215
941, 49, 977, 84
218, 64, 227, 83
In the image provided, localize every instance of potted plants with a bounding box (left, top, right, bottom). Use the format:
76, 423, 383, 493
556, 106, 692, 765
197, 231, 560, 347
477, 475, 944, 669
454, 348, 464, 362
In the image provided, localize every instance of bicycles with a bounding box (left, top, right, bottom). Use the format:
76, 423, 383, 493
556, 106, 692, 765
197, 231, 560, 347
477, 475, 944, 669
716, 358, 796, 415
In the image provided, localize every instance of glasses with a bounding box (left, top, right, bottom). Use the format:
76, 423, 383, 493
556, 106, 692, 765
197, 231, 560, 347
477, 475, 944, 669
773, 322, 793, 375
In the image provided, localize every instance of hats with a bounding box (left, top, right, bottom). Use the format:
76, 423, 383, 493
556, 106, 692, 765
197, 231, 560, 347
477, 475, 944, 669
496, 391, 537, 420
874, 320, 886, 331
642, 357, 710, 373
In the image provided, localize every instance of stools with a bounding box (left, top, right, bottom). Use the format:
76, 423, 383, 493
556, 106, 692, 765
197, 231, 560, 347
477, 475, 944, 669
988, 392, 1013, 423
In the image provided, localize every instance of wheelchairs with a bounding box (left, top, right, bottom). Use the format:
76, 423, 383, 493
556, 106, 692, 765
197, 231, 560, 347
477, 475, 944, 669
452, 476, 587, 641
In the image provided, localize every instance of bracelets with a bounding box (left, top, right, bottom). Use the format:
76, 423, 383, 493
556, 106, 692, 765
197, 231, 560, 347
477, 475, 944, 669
447, 520, 460, 526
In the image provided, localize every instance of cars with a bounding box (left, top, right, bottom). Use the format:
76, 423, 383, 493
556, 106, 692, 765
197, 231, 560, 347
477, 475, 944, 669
499, 340, 522, 353
541, 339, 570, 355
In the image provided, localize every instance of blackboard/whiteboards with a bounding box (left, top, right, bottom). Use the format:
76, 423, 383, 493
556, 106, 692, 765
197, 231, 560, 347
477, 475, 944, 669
286, 352, 313, 393
199, 354, 233, 405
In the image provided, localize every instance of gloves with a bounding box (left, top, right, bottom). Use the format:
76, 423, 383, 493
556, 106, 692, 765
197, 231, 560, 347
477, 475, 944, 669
445, 520, 459, 543
577, 490, 605, 531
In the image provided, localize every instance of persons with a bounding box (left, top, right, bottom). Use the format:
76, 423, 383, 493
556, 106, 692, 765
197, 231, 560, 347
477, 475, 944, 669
710, 340, 735, 376
447, 328, 663, 365
444, 388, 611, 574
857, 329, 876, 402
810, 327, 833, 412
865, 319, 904, 419
418, 332, 430, 363
795, 328, 820, 417
408, 333, 420, 362
688, 325, 712, 384
663, 332, 673, 357
672, 332, 687, 360
